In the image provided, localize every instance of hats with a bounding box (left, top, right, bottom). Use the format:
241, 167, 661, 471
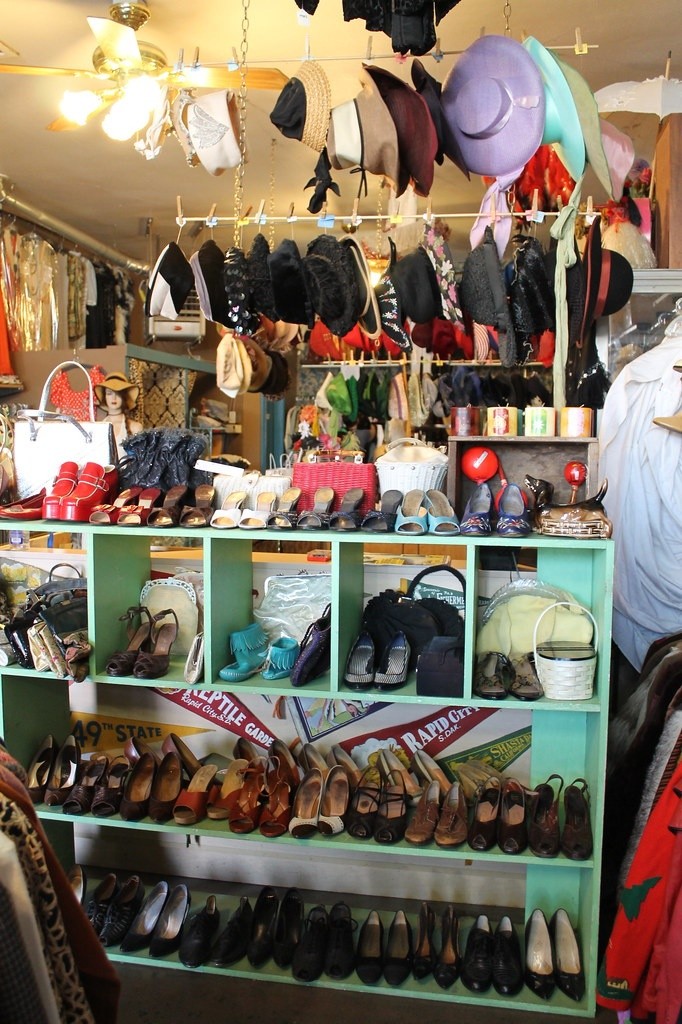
269, 35, 635, 203
188, 85, 249, 178
145, 215, 634, 427
94, 372, 139, 412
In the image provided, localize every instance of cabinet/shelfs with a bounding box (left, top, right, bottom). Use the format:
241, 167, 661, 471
0, 512, 615, 1020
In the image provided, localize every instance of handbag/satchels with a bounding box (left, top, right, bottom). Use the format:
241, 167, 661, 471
364, 565, 466, 662
290, 603, 331, 687
374, 437, 448, 500
4, 563, 92, 683
292, 451, 377, 519
212, 470, 291, 511
118, 428, 213, 507
14, 361, 119, 499
184, 632, 206, 685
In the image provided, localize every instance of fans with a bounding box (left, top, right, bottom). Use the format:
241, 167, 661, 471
0, 0, 290, 132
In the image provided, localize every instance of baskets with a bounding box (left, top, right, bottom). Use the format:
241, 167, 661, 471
533, 602, 599, 701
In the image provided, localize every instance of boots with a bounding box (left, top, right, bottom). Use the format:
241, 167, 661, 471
219, 622, 269, 682
262, 637, 300, 680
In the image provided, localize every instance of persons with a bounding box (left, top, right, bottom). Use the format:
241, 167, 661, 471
100, 379, 142, 470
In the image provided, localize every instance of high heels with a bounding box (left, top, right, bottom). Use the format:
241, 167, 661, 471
106, 606, 154, 679
343, 630, 374, 691
374, 631, 410, 690
65, 863, 585, 1002
26, 733, 593, 861
133, 609, 179, 678
42, 462, 531, 537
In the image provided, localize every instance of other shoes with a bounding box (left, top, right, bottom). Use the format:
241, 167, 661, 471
508, 653, 543, 701
473, 651, 516, 700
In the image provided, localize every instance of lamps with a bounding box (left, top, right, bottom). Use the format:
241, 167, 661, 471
59, 0, 162, 142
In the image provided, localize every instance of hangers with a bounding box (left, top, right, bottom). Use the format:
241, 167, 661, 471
0, 185, 136, 288
608, 296, 682, 434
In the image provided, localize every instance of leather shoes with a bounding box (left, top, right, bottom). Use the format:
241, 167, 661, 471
0, 487, 46, 520
